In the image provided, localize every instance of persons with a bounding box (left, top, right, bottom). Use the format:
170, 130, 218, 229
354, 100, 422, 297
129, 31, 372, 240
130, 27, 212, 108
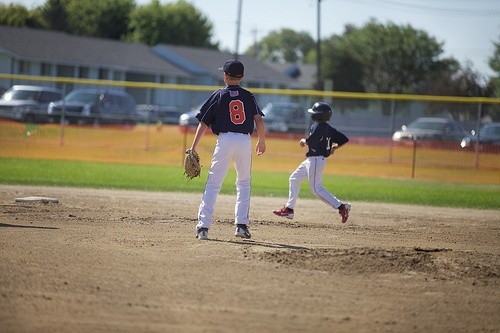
273, 102, 352, 224
184, 60, 266, 240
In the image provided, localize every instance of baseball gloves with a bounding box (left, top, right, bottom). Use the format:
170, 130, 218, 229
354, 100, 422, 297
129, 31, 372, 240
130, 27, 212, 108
183, 147, 203, 182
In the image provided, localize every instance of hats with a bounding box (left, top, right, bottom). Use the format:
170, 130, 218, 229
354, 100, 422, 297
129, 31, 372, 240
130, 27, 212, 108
216, 59, 245, 78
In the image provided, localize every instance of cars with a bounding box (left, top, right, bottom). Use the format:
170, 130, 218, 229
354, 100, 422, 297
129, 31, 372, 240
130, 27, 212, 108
0, 84, 65, 123
178, 101, 206, 126
392, 117, 468, 143
257, 102, 308, 133
459, 122, 500, 152
46, 89, 147, 129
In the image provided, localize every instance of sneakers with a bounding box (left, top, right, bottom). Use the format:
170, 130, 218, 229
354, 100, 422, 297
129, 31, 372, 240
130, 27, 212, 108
273, 207, 294, 220
195, 228, 209, 240
234, 225, 251, 239
338, 203, 352, 223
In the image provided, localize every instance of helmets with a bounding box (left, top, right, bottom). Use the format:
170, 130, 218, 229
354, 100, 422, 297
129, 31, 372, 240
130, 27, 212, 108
308, 101, 332, 121
